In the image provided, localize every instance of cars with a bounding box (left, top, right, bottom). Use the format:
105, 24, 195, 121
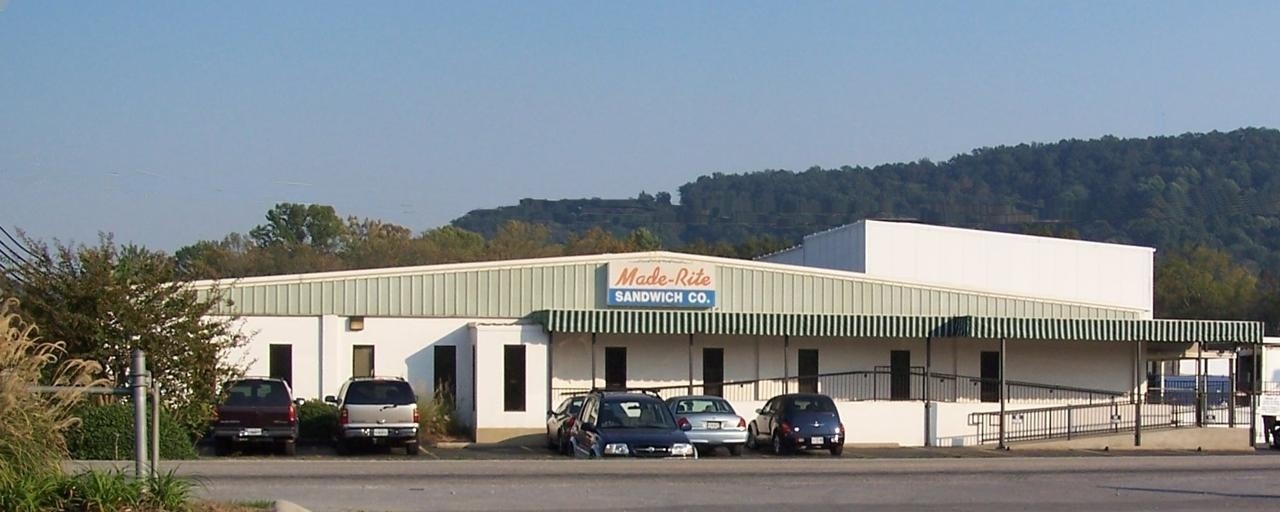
209, 375, 305, 457
325, 377, 421, 456
546, 390, 846, 460
